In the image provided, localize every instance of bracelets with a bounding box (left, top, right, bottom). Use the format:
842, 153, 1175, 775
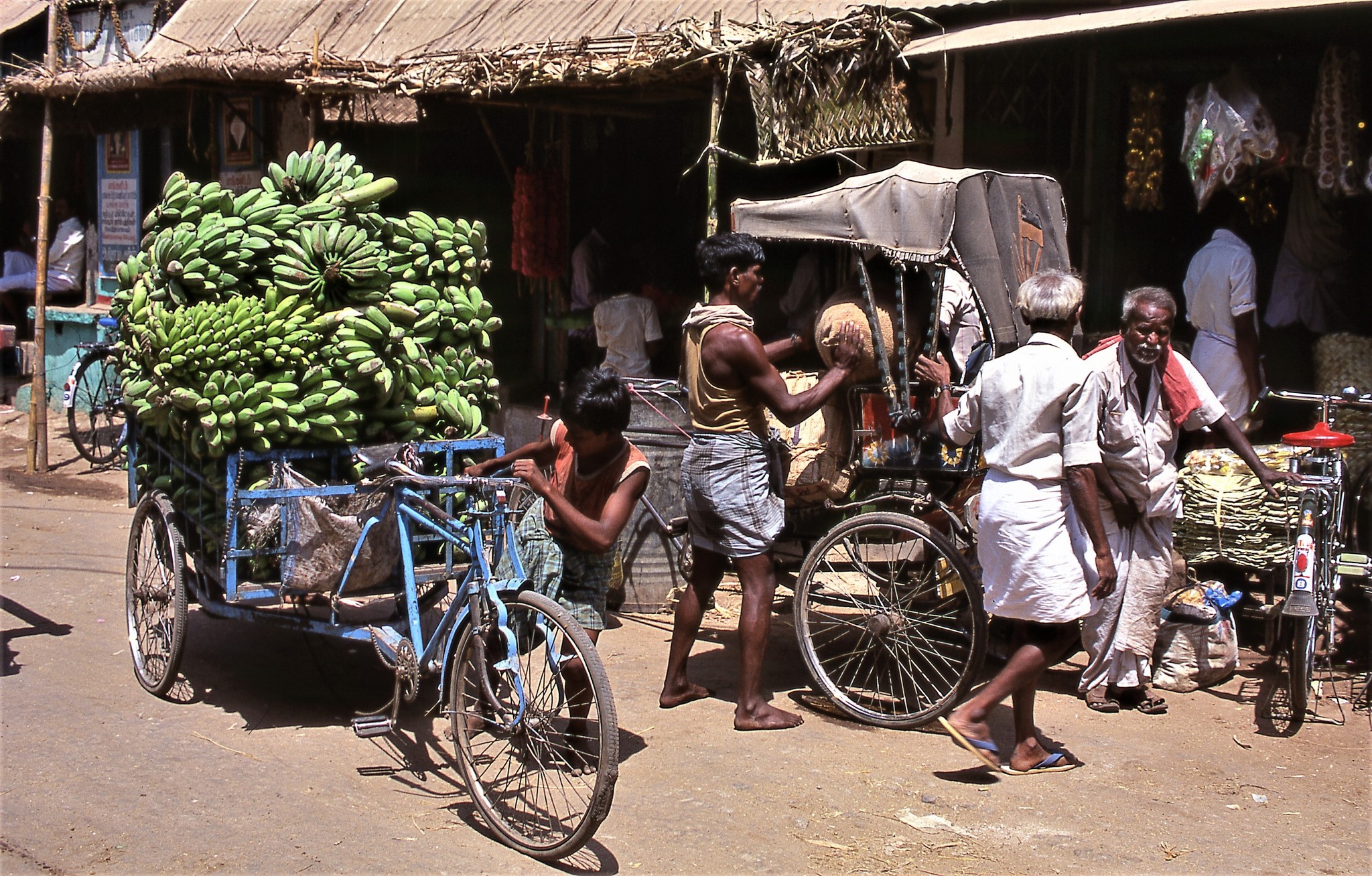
790, 334, 800, 354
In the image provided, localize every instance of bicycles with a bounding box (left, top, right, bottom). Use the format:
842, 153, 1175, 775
1249, 386, 1372, 726
64, 318, 135, 470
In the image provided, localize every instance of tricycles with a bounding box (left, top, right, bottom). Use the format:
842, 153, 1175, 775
726, 166, 1073, 726
125, 405, 617, 857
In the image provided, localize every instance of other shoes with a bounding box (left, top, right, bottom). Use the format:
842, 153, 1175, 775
1086, 682, 1169, 713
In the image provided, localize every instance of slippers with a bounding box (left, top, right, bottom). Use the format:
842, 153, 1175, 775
937, 715, 1002, 773
1000, 748, 1079, 775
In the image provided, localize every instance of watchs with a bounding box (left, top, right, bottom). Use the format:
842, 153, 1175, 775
933, 385, 951, 399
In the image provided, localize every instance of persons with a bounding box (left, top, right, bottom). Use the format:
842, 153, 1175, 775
592, 266, 667, 380
0, 193, 85, 294
913, 266, 1116, 777
464, 367, 650, 752
767, 247, 824, 352
1178, 183, 1273, 442
11, 204, 38, 251
1075, 284, 1300, 715
940, 266, 983, 385
565, 207, 627, 389
659, 228, 864, 733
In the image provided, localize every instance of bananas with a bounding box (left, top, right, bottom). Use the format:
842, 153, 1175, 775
109, 140, 503, 581
